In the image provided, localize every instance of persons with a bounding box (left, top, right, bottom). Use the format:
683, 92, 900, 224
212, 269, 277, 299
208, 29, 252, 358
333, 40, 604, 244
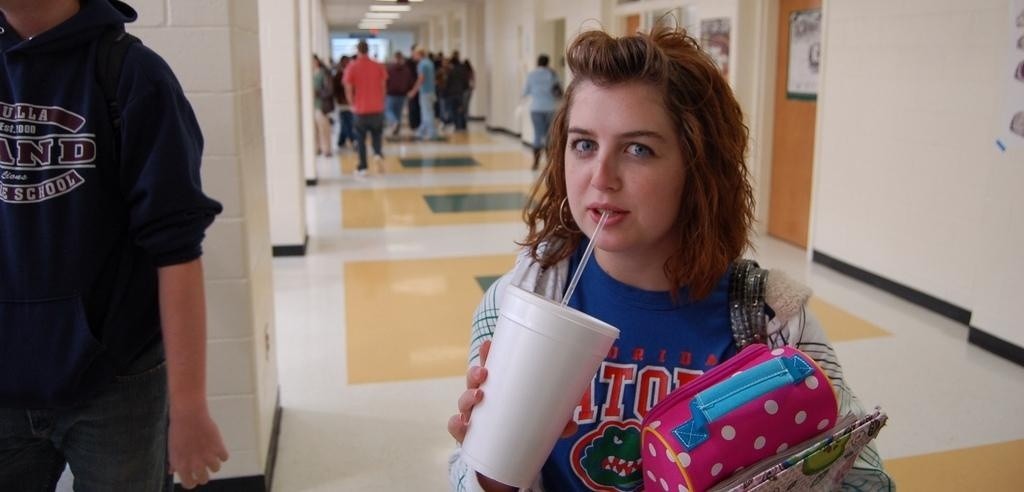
428, 49, 475, 130
342, 39, 390, 174
518, 53, 563, 171
0, 1, 230, 492
445, 10, 899, 490
408, 44, 439, 140
407, 43, 421, 129
335, 56, 358, 150
383, 51, 413, 140
314, 54, 336, 157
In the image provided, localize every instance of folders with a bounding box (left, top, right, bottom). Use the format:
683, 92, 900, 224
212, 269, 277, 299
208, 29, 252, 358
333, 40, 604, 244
730, 412, 886, 492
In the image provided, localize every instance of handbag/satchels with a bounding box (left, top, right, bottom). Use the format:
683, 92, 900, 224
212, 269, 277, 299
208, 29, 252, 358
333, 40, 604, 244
549, 68, 564, 98
